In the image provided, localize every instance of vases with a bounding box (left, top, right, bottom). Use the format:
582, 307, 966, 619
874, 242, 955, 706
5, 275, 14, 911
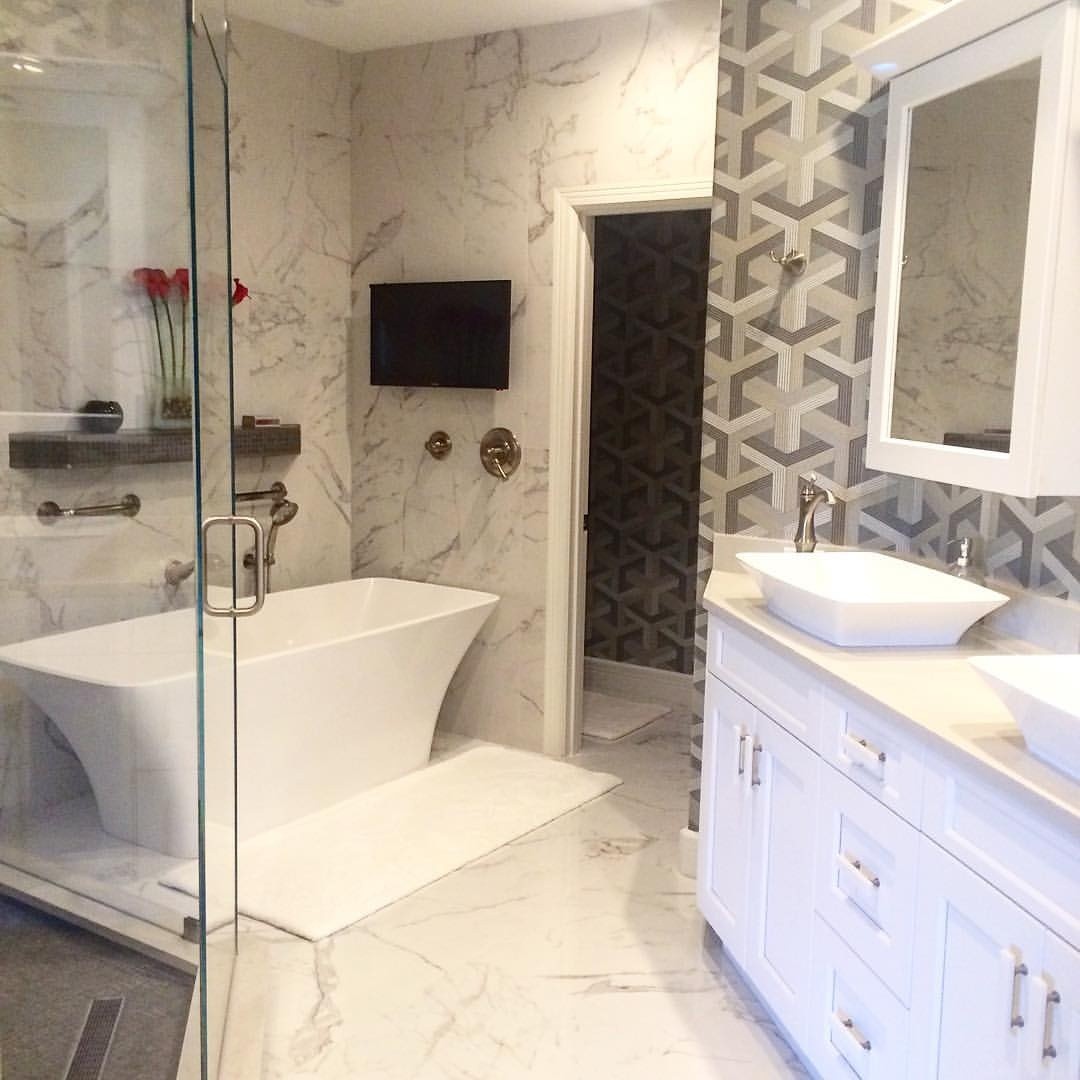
146, 301, 196, 429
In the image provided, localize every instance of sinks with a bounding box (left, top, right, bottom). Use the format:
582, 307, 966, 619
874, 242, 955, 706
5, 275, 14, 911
735, 547, 1012, 651
967, 656, 1079, 776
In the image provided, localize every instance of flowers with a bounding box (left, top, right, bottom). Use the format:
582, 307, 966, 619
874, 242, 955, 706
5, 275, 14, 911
134, 267, 250, 403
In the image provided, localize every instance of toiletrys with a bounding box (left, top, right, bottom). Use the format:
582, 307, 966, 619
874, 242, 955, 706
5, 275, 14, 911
945, 536, 990, 627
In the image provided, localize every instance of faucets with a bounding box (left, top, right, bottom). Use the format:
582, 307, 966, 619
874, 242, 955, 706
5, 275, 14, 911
792, 472, 839, 552
164, 553, 226, 586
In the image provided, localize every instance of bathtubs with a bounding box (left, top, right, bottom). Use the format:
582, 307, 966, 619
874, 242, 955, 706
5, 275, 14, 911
1, 571, 506, 857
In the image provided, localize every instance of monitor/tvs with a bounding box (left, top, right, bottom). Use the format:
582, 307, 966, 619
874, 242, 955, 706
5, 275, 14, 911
370, 279, 510, 390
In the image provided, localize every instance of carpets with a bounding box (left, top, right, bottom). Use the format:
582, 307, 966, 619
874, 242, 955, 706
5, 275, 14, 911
159, 739, 623, 937
581, 691, 672, 740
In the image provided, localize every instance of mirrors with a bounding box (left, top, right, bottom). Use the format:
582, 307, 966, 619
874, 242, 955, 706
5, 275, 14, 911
865, 2, 1071, 501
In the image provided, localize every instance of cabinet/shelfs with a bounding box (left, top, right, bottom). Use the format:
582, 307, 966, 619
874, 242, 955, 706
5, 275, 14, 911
694, 616, 1080, 1080
